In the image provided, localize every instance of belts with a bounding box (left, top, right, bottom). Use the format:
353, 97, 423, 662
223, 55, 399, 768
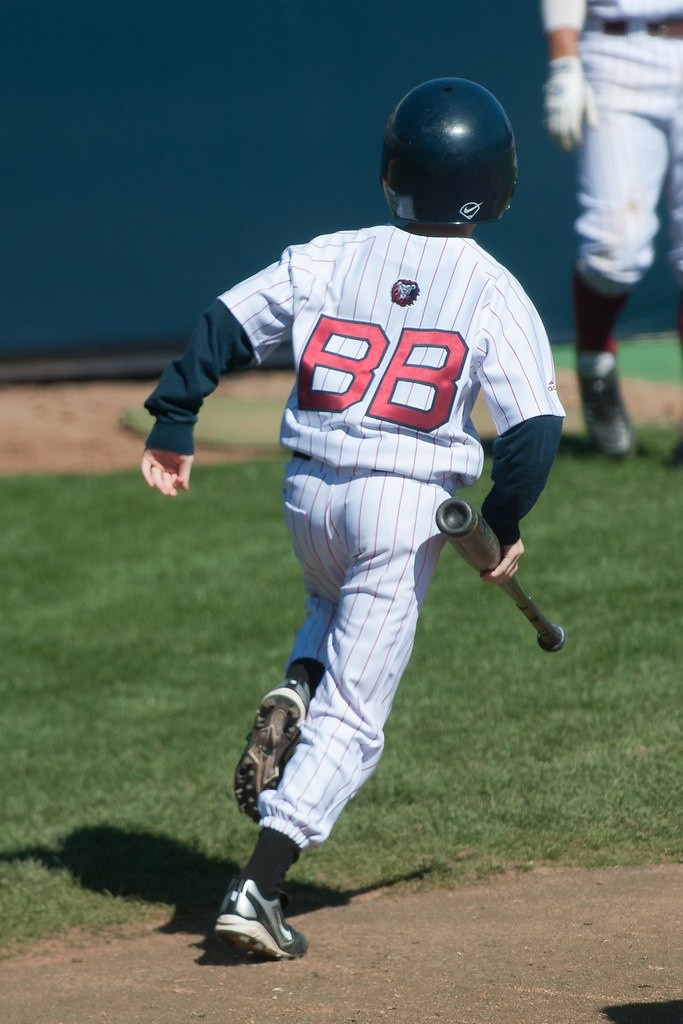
604, 13, 683, 40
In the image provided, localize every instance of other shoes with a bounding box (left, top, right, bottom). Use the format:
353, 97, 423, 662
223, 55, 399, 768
577, 336, 634, 457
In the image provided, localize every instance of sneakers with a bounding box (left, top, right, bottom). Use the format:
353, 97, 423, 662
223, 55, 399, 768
234, 678, 311, 824
214, 878, 310, 957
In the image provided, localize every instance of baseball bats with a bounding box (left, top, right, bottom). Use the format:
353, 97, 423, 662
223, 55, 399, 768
434, 495, 567, 653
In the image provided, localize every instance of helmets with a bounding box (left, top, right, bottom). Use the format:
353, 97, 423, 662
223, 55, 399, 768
381, 77, 518, 225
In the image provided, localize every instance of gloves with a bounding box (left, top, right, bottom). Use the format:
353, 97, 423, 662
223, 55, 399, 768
543, 56, 600, 151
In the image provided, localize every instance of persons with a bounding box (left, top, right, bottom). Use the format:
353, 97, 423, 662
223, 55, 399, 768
139, 78, 564, 959
540, 0, 683, 466
